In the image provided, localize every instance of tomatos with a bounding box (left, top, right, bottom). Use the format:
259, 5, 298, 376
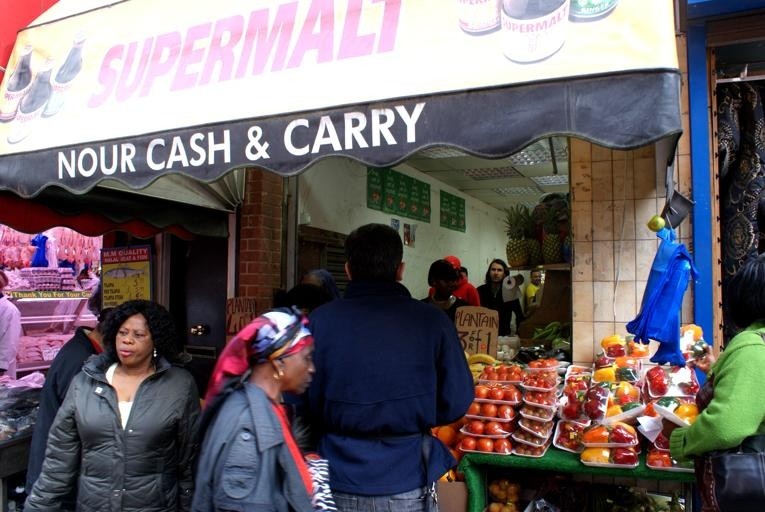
464, 359, 559, 455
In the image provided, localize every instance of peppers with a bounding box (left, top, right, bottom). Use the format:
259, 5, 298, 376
556, 324, 707, 468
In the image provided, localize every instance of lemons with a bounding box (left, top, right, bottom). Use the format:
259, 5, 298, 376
648, 216, 666, 231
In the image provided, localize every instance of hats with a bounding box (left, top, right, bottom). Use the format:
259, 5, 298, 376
428, 260, 460, 287
444, 255, 462, 270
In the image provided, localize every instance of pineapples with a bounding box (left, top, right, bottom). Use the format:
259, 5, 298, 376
504, 203, 570, 268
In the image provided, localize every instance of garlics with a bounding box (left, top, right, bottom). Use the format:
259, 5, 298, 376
496, 335, 517, 360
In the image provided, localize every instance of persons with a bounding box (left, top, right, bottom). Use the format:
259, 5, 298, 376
284, 268, 340, 452
669, 254, 765, 512
421, 256, 545, 335
22, 307, 116, 502
284, 223, 474, 511
22, 300, 202, 512
0, 269, 21, 381
191, 305, 337, 512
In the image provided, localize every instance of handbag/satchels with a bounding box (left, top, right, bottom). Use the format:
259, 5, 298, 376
694, 443, 765, 511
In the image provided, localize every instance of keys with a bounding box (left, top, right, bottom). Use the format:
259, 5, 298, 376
420, 482, 438, 511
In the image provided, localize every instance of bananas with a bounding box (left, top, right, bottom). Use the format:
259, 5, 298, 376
463, 351, 499, 383
